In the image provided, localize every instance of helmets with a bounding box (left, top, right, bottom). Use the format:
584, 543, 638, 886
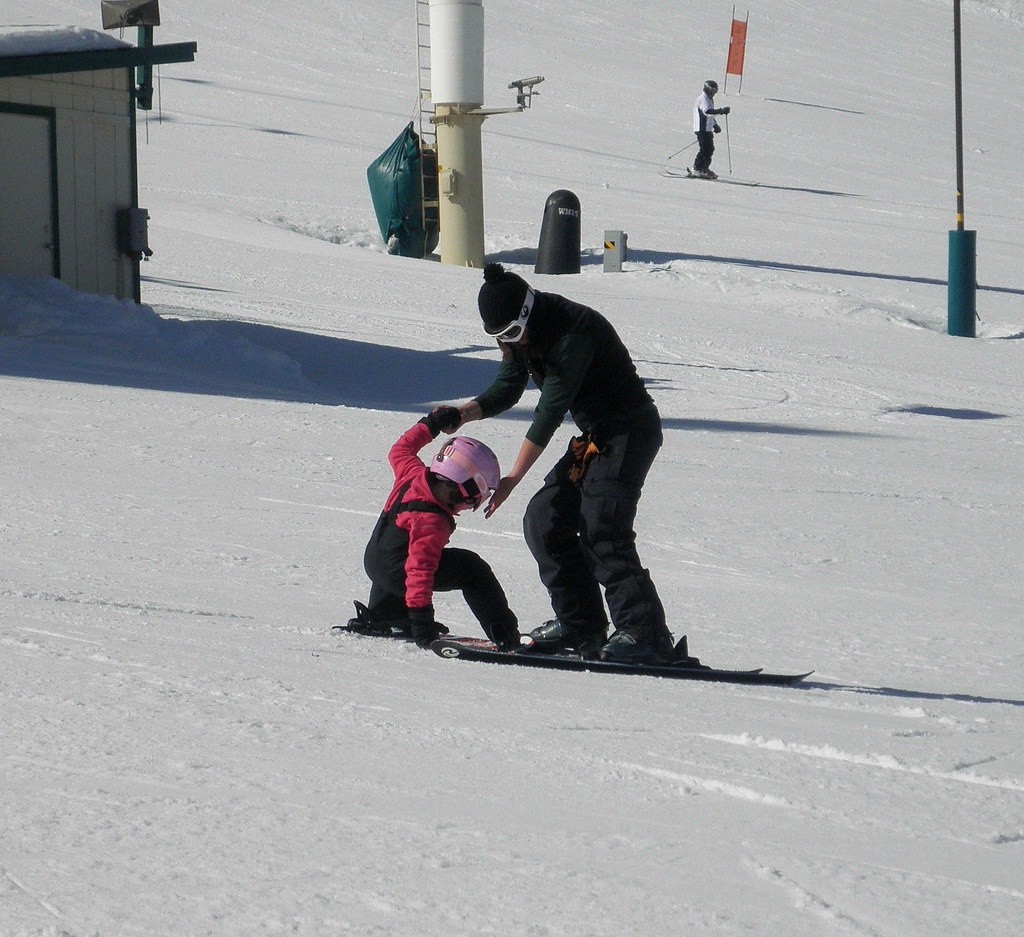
430, 436, 500, 511
703, 80, 719, 99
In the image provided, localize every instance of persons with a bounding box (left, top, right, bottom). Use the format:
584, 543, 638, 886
691, 80, 730, 179
439, 264, 675, 663
364, 406, 523, 653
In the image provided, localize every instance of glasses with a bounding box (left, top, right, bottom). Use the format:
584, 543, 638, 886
481, 284, 536, 342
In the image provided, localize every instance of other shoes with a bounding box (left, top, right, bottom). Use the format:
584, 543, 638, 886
529, 618, 608, 651
601, 624, 675, 661
499, 636, 534, 655
692, 165, 717, 179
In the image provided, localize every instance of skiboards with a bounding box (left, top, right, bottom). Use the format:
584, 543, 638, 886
431, 630, 817, 689
657, 167, 762, 188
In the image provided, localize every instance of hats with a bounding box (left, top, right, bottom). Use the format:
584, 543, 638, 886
478, 263, 536, 331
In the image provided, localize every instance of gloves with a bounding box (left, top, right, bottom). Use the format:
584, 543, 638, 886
712, 124, 721, 133
720, 107, 730, 115
417, 405, 461, 439
409, 603, 449, 651
